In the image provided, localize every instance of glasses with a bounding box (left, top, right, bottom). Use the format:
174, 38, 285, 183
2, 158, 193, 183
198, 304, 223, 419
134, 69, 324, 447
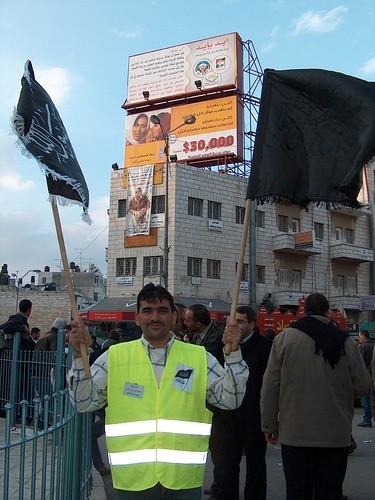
235, 320, 248, 328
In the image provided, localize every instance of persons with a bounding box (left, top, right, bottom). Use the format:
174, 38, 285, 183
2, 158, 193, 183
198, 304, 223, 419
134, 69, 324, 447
260, 294, 372, 500
0, 299, 374, 500
66, 282, 249, 500
126, 112, 170, 147
128, 186, 150, 224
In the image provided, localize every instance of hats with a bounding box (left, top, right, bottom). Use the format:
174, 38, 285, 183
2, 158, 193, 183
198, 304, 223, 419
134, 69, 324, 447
109, 330, 119, 341
114, 328, 123, 334
52, 317, 67, 329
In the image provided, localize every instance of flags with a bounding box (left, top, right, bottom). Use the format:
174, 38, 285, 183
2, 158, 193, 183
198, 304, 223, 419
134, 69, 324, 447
245, 67, 375, 214
11, 59, 92, 226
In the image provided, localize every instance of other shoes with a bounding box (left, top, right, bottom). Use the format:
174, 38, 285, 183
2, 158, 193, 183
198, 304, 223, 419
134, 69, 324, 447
357, 421, 372, 427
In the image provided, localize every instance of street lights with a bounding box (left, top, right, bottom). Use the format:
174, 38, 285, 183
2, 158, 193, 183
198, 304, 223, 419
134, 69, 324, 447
149, 115, 197, 290
11, 270, 19, 314
